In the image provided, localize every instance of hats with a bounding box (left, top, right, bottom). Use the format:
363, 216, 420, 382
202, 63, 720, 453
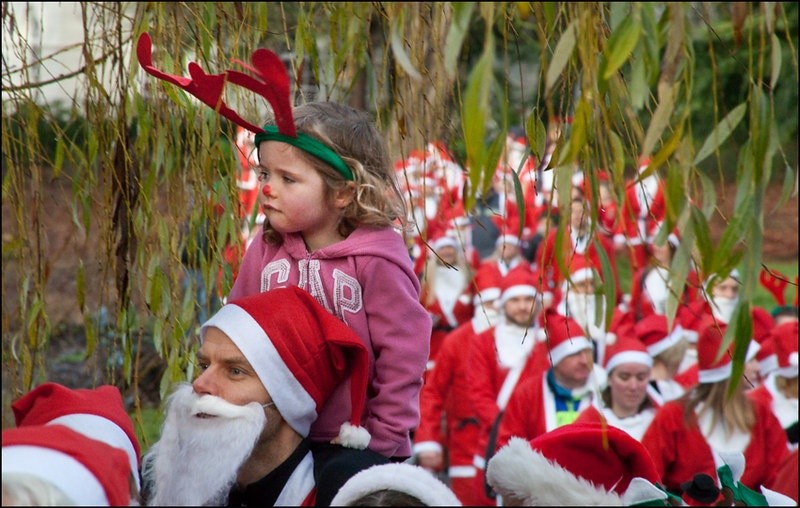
678, 297, 713, 343
329, 463, 462, 507
12, 382, 141, 492
696, 316, 760, 384
486, 421, 668, 505
635, 312, 685, 357
390, 132, 681, 255
200, 282, 371, 450
562, 255, 593, 292
459, 263, 503, 306
602, 303, 653, 372
2, 423, 131, 506
702, 269, 742, 302
749, 309, 799, 378
624, 265, 671, 313
537, 307, 594, 366
491, 263, 543, 308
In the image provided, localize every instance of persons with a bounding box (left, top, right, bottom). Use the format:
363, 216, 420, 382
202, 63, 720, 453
138, 33, 430, 507
1, 32, 799, 507
139, 285, 371, 507
